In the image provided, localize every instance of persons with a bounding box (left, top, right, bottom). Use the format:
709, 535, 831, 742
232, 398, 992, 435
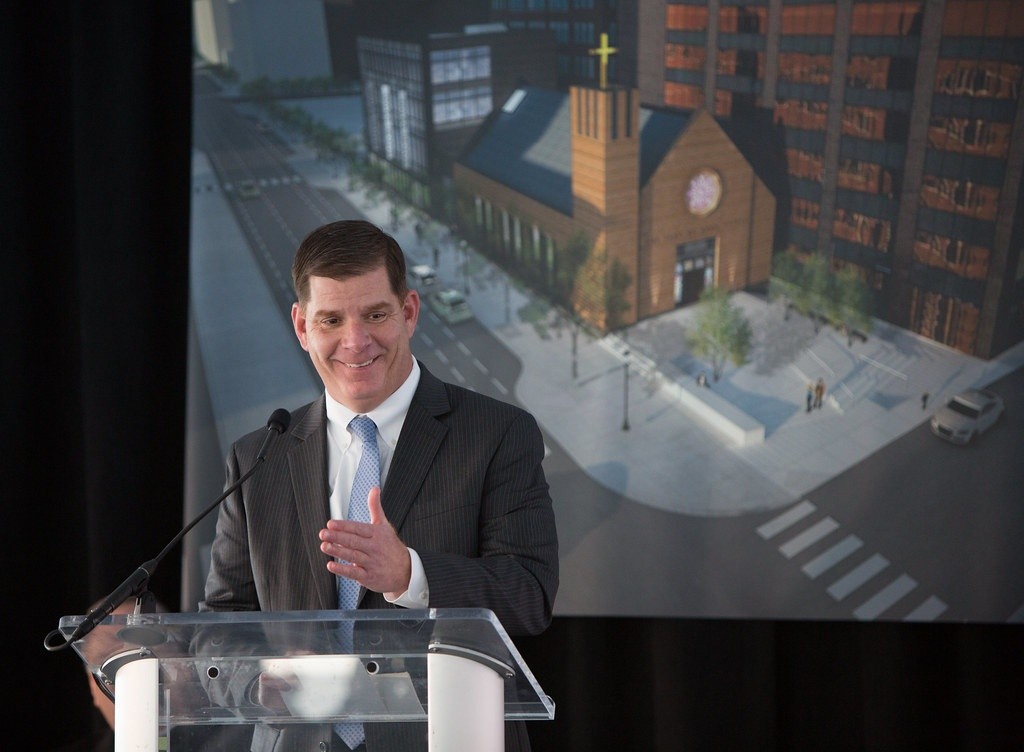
385, 205, 444, 270
920, 391, 926, 410
189, 218, 561, 752
804, 377, 826, 414
692, 368, 708, 387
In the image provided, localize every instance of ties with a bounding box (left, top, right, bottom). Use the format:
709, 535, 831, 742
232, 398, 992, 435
334, 415, 381, 750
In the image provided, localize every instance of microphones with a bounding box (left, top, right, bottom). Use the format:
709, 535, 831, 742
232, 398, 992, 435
257, 408, 292, 464
